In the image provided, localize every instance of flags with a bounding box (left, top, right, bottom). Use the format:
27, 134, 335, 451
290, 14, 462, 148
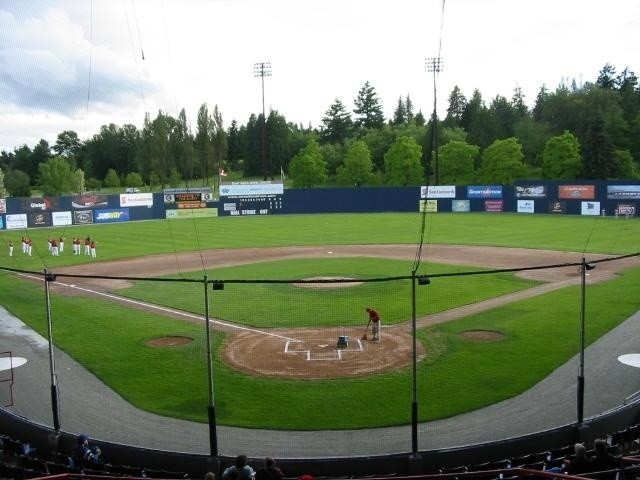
218, 167, 228, 177
281, 168, 286, 180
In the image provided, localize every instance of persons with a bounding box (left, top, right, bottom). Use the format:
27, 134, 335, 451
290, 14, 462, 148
546, 439, 616, 475
222, 455, 255, 480
9, 233, 97, 259
255, 457, 285, 479
367, 308, 380, 341
205, 472, 215, 479
54, 434, 101, 467
303, 474, 312, 479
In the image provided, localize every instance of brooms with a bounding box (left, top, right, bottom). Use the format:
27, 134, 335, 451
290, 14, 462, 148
361, 321, 371, 340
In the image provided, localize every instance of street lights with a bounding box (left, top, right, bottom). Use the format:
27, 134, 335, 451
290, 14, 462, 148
424, 56, 445, 186
253, 60, 273, 181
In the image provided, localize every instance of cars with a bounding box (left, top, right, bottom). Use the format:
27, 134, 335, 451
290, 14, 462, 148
125, 188, 139, 194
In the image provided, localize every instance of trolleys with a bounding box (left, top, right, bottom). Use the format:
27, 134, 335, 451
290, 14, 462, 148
336, 335, 349, 347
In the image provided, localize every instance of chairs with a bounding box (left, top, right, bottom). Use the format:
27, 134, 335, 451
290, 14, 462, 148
1, 394, 640, 479
